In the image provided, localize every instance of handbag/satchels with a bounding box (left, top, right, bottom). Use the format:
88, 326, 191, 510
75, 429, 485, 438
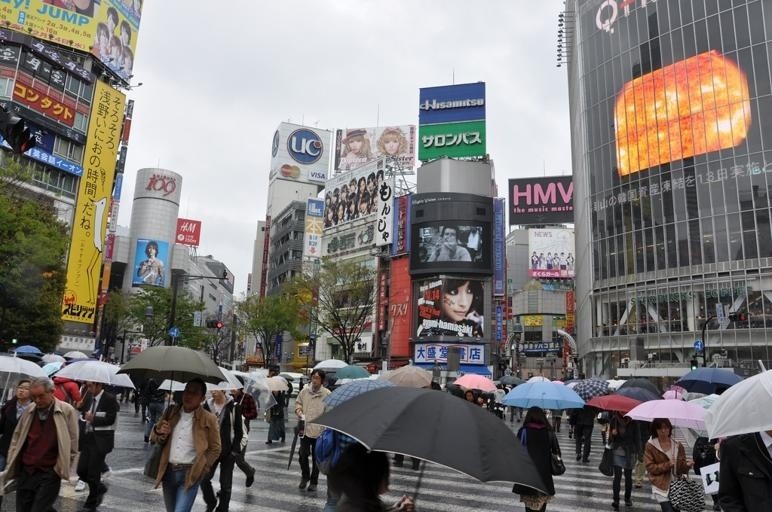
670, 478, 705, 512
314, 428, 340, 474
599, 449, 614, 476
144, 444, 162, 478
231, 416, 249, 452
699, 462, 720, 495
552, 453, 565, 475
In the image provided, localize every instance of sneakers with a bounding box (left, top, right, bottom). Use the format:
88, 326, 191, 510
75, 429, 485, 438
299, 478, 308, 488
307, 485, 317, 490
76, 466, 112, 508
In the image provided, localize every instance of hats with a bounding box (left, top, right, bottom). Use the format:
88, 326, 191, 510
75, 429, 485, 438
343, 130, 367, 139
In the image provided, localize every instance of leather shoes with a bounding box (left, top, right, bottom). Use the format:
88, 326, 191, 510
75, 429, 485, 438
246, 475, 253, 487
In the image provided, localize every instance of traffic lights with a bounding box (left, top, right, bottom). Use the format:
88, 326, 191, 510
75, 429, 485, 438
207, 321, 225, 328
691, 360, 698, 370
728, 313, 748, 321
0, 104, 36, 155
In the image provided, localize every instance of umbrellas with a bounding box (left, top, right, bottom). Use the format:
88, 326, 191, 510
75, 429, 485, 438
306, 385, 550, 511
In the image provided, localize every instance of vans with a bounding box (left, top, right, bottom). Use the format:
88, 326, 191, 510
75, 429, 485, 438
276, 371, 311, 397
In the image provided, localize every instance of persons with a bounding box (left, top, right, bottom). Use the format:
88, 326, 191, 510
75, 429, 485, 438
70, 0, 99, 18
340, 129, 373, 167
376, 127, 409, 157
94, 1, 143, 73
529, 250, 574, 270
423, 382, 771, 511
429, 224, 472, 261
137, 239, 167, 287
324, 172, 377, 227
1, 370, 414, 511
438, 279, 483, 321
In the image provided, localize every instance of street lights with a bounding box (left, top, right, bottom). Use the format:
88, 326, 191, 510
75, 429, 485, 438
285, 352, 294, 362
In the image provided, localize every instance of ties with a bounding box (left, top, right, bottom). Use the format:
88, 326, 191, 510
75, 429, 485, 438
92, 398, 95, 414
